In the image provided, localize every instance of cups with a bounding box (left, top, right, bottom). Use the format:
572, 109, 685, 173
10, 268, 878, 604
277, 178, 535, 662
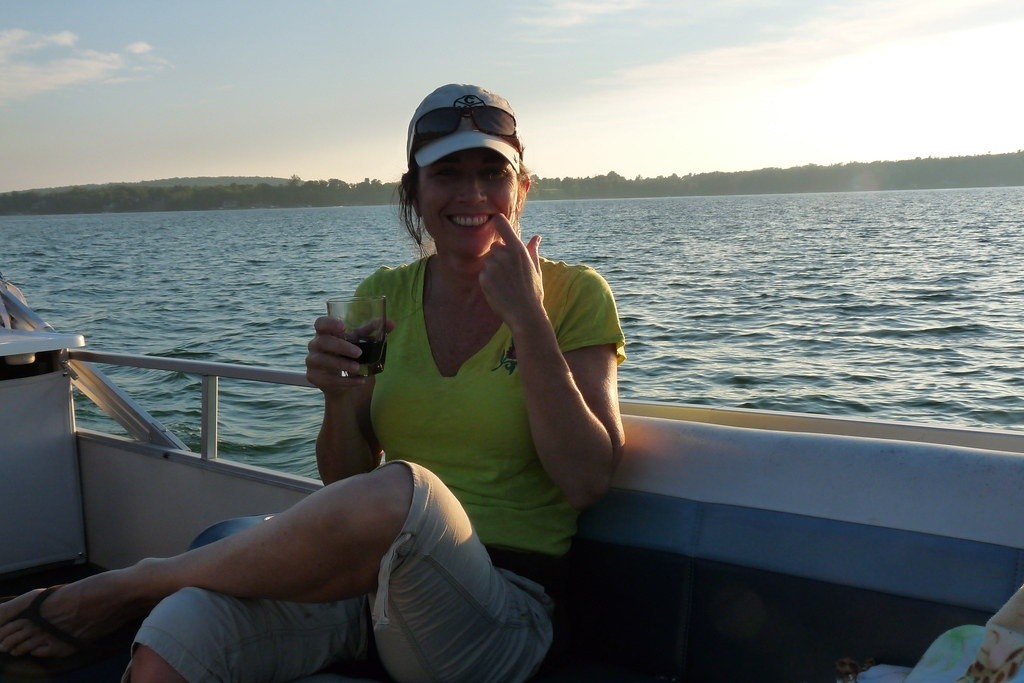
326, 296, 388, 379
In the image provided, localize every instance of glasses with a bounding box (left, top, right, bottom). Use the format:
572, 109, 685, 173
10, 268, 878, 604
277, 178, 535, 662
409, 106, 516, 171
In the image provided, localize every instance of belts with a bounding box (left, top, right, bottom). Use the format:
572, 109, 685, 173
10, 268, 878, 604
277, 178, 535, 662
480, 548, 569, 599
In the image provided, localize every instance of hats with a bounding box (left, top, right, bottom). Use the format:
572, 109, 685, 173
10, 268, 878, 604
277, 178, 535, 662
406, 83, 523, 174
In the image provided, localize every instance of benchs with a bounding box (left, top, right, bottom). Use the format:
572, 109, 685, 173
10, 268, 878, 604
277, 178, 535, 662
190, 412, 1023, 683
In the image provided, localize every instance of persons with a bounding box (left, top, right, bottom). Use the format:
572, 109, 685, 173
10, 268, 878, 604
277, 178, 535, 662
0, 84, 628, 681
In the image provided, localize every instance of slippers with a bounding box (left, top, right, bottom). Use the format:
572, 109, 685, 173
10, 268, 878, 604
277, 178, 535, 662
0, 583, 144, 676
